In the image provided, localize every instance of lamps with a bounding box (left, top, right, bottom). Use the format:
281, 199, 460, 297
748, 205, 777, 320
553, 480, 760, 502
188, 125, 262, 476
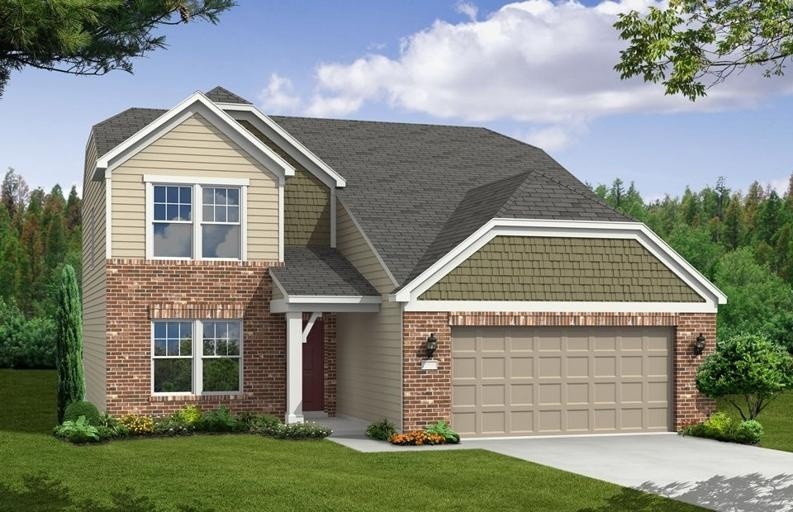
694, 331, 707, 357
420, 334, 439, 370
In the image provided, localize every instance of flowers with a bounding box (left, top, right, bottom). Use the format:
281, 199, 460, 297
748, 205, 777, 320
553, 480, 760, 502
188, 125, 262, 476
393, 430, 444, 445
